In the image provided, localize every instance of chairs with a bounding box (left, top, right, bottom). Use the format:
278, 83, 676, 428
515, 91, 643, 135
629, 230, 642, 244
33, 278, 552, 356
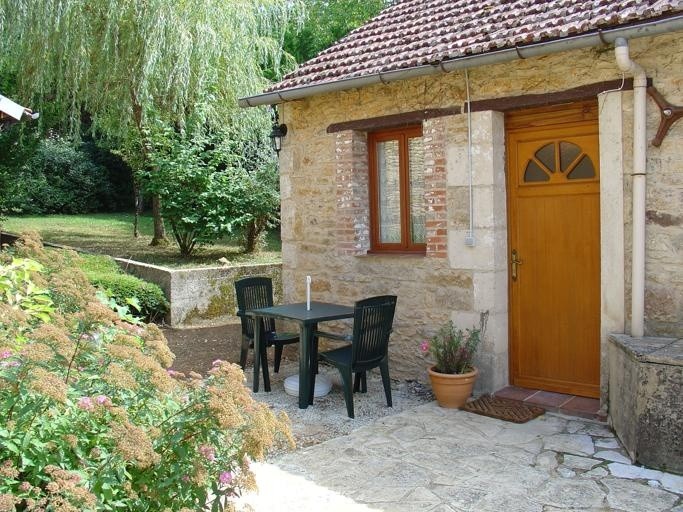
234, 276, 397, 419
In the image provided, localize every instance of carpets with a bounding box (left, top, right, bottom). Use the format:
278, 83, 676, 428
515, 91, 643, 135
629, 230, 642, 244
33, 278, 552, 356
459, 395, 545, 423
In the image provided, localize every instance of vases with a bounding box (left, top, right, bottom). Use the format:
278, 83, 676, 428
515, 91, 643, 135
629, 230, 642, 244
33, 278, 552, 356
425, 364, 478, 409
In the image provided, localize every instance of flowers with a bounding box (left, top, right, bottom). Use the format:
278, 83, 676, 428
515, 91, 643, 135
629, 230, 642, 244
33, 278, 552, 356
418, 321, 481, 374
